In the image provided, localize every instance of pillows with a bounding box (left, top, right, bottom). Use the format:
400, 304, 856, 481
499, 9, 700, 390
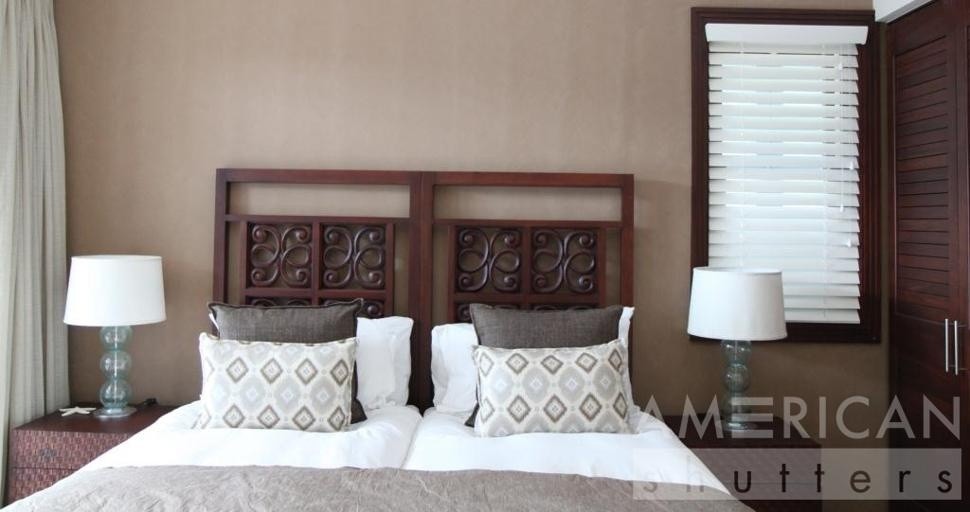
189, 296, 640, 436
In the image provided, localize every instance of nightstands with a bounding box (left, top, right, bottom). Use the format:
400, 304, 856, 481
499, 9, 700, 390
5, 402, 177, 506
659, 414, 822, 510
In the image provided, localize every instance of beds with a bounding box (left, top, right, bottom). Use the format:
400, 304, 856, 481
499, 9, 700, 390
0, 166, 758, 511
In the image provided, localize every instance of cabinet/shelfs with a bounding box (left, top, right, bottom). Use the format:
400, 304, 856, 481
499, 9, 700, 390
884, 0, 969, 512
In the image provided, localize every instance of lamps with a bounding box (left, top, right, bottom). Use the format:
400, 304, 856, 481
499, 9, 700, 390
62, 254, 168, 419
686, 267, 789, 434
